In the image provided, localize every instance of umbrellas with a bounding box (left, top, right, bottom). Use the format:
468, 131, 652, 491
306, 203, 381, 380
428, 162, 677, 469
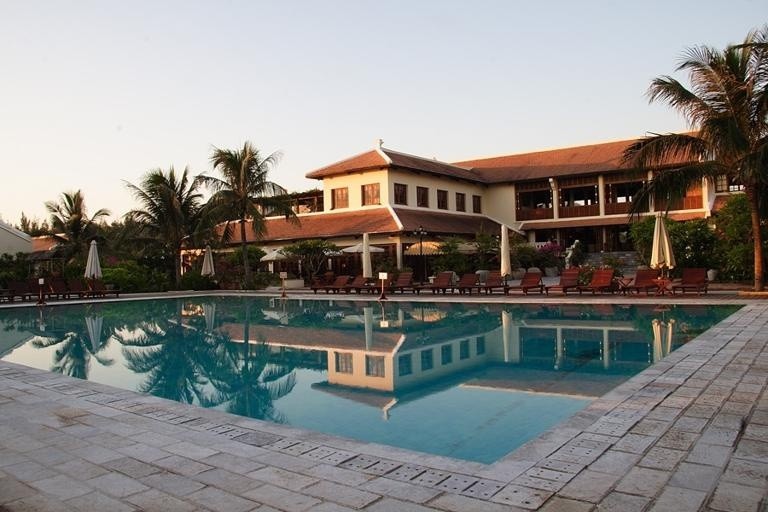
650, 213, 677, 278
200, 244, 216, 289
201, 301, 217, 330
84, 240, 103, 285
84, 314, 104, 354
651, 312, 676, 360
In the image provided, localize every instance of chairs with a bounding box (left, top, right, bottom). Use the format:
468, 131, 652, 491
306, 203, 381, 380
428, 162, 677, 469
0, 278, 121, 304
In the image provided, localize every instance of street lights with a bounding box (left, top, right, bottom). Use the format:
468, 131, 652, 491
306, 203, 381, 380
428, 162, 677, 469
414, 226, 427, 286
